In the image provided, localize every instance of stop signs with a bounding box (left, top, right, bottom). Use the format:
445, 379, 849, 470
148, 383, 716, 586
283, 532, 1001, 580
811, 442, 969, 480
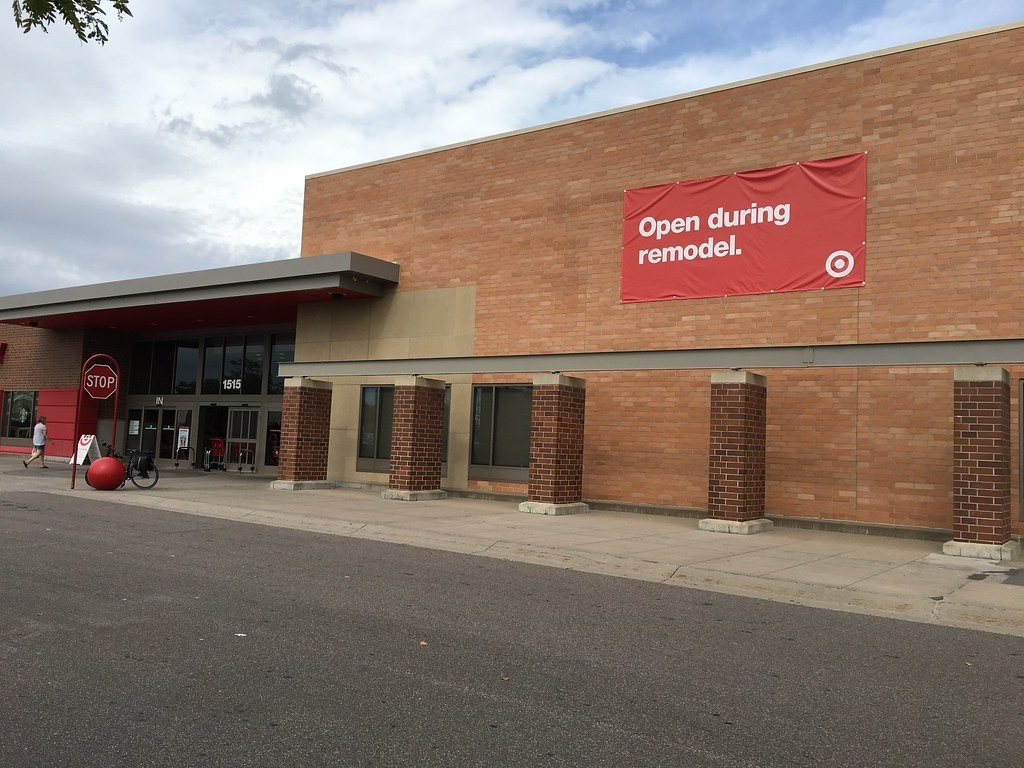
83, 364, 117, 400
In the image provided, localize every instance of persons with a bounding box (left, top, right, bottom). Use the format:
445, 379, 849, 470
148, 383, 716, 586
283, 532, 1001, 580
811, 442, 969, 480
23, 416, 54, 469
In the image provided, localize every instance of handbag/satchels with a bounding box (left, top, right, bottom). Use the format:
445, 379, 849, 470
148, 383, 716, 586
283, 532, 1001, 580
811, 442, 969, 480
30, 447, 40, 458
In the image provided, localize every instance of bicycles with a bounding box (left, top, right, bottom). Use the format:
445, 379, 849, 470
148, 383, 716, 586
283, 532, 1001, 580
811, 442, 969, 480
84, 440, 159, 491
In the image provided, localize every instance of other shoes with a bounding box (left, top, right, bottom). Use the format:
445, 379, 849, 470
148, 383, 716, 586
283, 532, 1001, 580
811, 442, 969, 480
23, 460, 28, 468
40, 465, 49, 468
